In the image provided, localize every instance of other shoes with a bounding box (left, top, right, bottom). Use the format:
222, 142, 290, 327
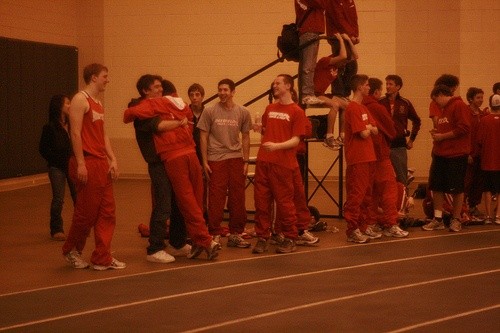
53, 232, 65, 240
485, 217, 491, 223
495, 216, 500, 224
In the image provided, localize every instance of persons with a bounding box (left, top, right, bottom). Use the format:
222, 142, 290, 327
185, 82, 210, 227
294, 0, 326, 105
245, 73, 319, 253
197, 79, 252, 248
421, 74, 500, 231
379, 75, 422, 225
123, 73, 221, 263
326, 0, 359, 104
361, 78, 409, 238
61, 63, 127, 270
39, 94, 78, 241
313, 33, 359, 150
342, 74, 382, 243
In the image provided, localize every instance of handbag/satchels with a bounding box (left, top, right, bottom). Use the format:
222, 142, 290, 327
277, 22, 301, 63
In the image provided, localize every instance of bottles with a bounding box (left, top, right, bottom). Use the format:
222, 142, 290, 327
255, 112, 262, 138
325, 225, 339, 233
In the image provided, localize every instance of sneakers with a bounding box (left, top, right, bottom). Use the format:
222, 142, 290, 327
347, 228, 369, 243
336, 136, 344, 145
384, 225, 408, 238
364, 226, 382, 239
296, 230, 320, 244
421, 218, 445, 230
64, 250, 89, 268
323, 135, 341, 150
252, 239, 268, 253
276, 238, 296, 253
187, 246, 201, 259
302, 95, 325, 105
213, 235, 221, 246
172, 245, 192, 256
147, 250, 175, 263
206, 240, 220, 260
448, 219, 462, 231
227, 232, 250, 247
93, 257, 126, 270
